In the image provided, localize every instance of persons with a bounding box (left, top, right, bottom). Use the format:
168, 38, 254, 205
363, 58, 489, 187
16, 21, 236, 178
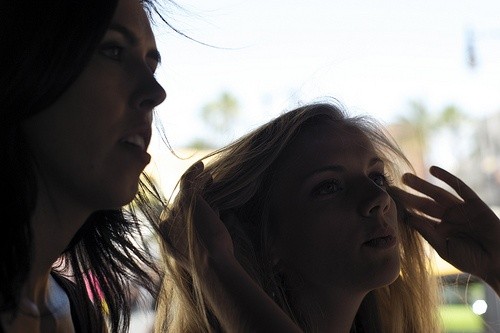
154, 103, 500, 333
0, 0, 167, 332
